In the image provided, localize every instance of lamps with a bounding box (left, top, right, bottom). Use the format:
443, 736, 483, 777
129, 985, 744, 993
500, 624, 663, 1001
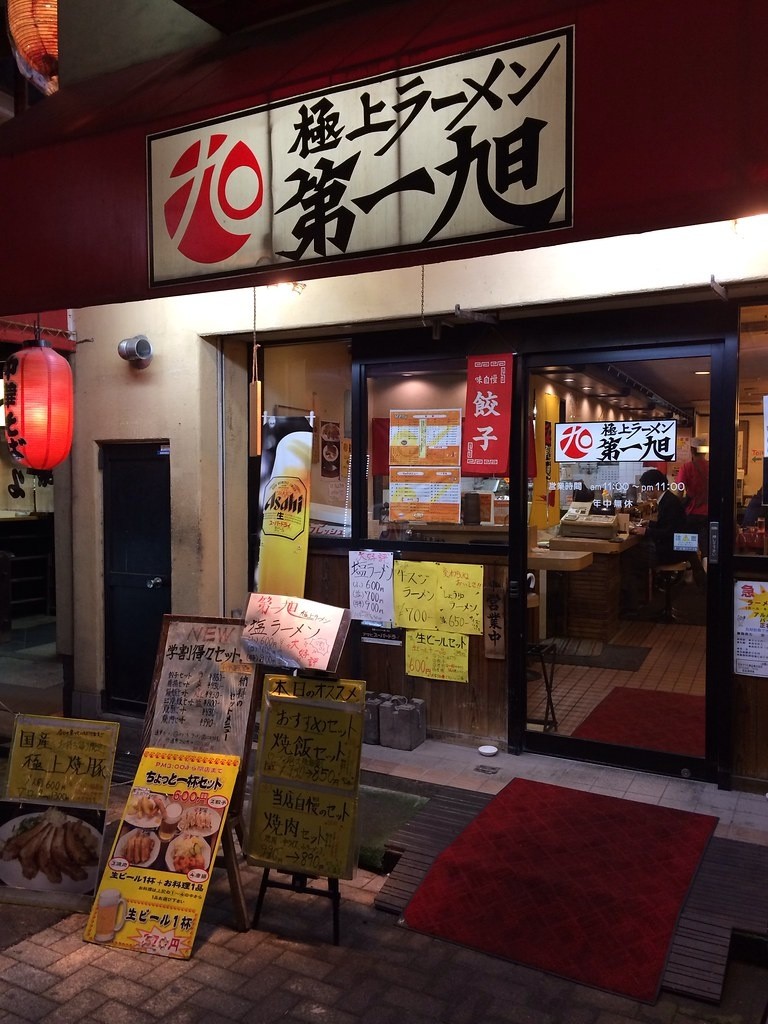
119, 336, 154, 371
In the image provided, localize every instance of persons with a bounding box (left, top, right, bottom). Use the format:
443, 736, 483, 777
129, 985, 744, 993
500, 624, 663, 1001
617, 469, 689, 622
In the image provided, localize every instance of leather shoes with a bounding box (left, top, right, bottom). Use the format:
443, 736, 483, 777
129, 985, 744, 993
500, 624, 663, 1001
643, 607, 668, 620
618, 607, 642, 618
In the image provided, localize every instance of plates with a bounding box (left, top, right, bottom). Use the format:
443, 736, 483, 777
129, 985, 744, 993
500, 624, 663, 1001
114, 829, 160, 867
165, 834, 211, 872
124, 794, 171, 827
0, 811, 102, 893
178, 806, 221, 837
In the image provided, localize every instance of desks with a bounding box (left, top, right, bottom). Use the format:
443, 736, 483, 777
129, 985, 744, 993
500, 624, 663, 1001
527, 552, 594, 643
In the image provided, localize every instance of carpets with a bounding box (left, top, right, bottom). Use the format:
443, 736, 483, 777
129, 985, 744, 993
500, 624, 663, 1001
397, 777, 720, 1004
571, 686, 706, 758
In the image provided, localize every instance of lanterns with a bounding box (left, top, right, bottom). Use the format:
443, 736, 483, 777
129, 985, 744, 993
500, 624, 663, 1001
0, 338, 75, 476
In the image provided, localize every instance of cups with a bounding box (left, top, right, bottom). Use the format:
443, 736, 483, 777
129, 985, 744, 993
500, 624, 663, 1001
95, 888, 127, 941
158, 802, 183, 842
